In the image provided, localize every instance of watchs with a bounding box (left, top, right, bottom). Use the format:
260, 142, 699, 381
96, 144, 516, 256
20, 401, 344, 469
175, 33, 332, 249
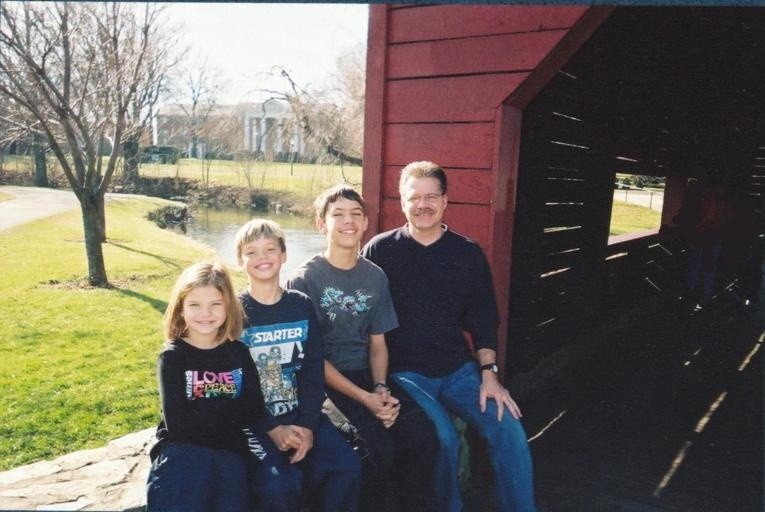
479, 363, 499, 375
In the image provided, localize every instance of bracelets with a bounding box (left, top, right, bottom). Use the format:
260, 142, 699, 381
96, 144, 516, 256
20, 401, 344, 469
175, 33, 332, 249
371, 383, 391, 391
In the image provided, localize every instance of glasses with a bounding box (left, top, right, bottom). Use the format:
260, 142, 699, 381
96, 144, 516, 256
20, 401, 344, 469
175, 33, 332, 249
403, 190, 448, 203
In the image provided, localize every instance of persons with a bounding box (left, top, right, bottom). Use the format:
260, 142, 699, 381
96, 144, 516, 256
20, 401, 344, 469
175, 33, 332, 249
288, 190, 439, 512
359, 161, 536, 511
145, 264, 263, 511
231, 218, 364, 511
